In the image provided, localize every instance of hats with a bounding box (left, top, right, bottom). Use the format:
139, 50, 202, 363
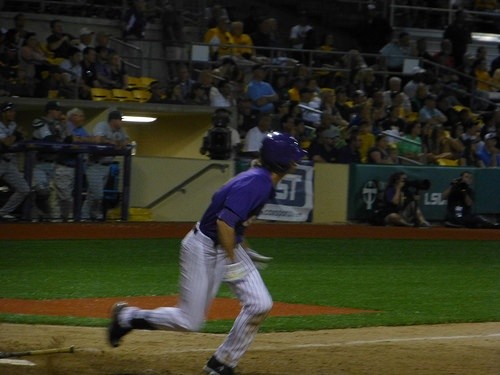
79, 28, 95, 37
95, 29, 113, 39
108, 110, 122, 120
43, 100, 67, 113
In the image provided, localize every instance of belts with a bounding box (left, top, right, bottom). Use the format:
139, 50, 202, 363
95, 160, 121, 166
55, 161, 78, 168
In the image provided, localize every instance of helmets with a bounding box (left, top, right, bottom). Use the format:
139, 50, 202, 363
260, 129, 310, 170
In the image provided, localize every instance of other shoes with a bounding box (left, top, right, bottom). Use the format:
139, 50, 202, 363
50, 218, 64, 223
67, 217, 75, 223
415, 221, 433, 227
1, 213, 17, 222
31, 218, 40, 224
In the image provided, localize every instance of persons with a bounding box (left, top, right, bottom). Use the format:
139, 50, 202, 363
383, 172, 430, 226
443, 171, 500, 230
0, 13, 128, 102
109, 133, 308, 375
30, 101, 73, 222
53, 107, 106, 222
79, 111, 128, 219
0, 100, 31, 219
119, 0, 500, 168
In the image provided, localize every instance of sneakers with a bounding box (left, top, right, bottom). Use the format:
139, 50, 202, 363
108, 302, 131, 348
203, 355, 238, 375
